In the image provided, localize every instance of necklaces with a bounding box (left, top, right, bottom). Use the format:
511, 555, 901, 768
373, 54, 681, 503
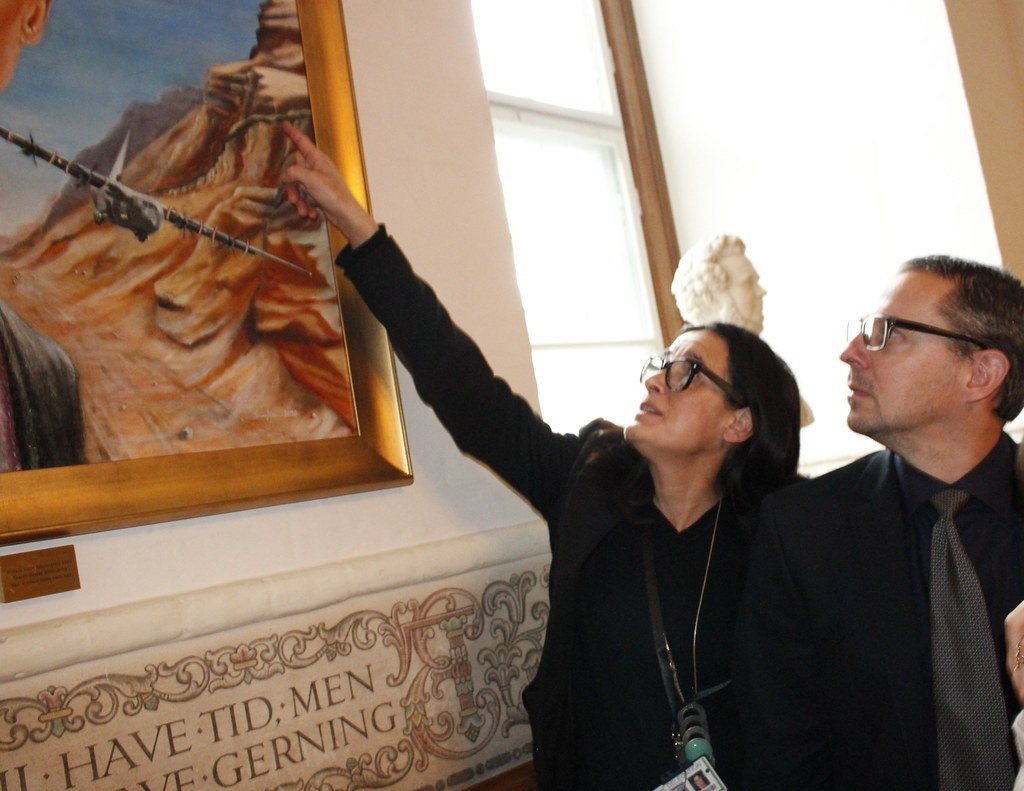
626, 471, 731, 766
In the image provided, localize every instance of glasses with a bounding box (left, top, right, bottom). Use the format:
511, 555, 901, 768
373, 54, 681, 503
847, 312, 988, 351
640, 355, 747, 408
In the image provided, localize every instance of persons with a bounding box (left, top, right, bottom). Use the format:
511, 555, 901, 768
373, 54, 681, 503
275, 115, 819, 788
672, 229, 815, 426
807, 252, 1020, 789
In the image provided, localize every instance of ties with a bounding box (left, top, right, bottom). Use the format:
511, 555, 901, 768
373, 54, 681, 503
926, 487, 1016, 791
